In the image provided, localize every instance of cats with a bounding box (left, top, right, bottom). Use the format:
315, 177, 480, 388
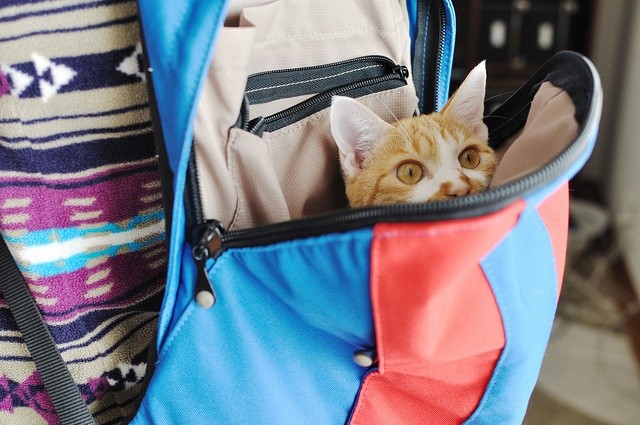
330, 58, 499, 209
